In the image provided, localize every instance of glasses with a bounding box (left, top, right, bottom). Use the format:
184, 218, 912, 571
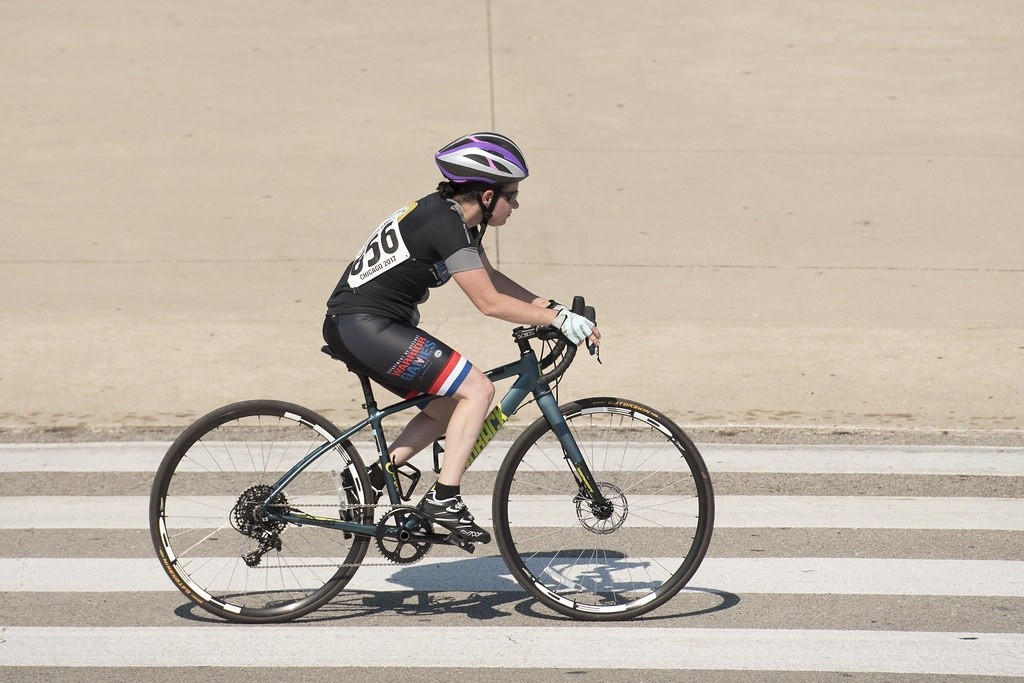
478, 188, 519, 204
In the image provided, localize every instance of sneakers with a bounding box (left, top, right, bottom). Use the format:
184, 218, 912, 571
420, 490, 491, 543
342, 466, 383, 534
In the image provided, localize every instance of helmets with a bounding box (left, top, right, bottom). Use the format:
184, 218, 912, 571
434, 132, 529, 184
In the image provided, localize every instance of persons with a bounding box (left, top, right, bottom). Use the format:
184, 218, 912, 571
323, 133, 601, 546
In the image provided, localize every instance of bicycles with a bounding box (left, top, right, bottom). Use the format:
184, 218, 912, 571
147, 298, 717, 624
